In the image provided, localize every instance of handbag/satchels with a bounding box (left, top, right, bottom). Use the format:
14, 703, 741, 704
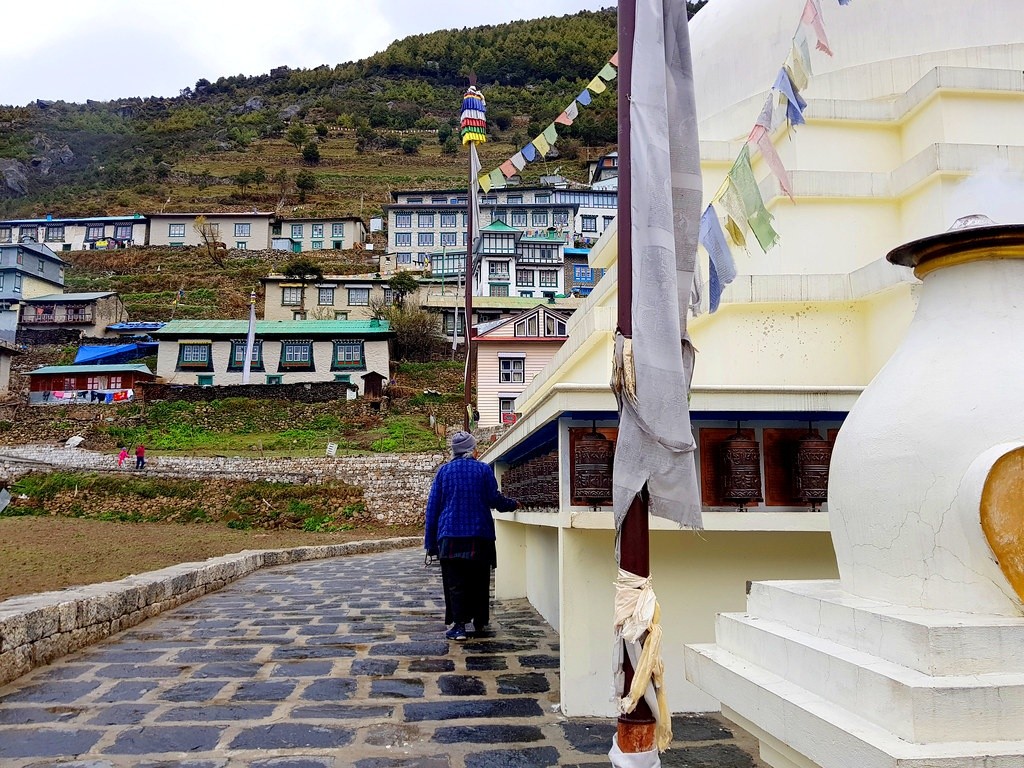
424, 515, 437, 566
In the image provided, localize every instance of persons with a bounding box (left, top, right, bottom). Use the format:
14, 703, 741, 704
135, 442, 145, 470
118, 447, 130, 467
423, 432, 523, 639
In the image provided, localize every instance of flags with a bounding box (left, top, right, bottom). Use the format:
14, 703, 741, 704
476, 0, 854, 313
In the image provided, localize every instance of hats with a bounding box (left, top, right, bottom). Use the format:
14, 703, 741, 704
451, 431, 476, 454
122, 447, 126, 449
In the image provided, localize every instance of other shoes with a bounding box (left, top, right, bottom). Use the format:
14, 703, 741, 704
445, 627, 466, 639
474, 624, 485, 631
